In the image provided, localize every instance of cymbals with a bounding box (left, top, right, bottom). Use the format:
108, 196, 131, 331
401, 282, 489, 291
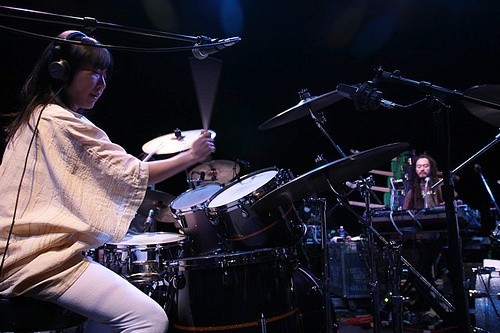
142, 130, 216, 154
249, 142, 410, 214
186, 160, 241, 187
259, 85, 356, 132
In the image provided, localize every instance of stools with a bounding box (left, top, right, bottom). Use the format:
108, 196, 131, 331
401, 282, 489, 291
0, 296, 87, 333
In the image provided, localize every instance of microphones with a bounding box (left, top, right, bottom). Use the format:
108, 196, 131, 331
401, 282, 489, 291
334, 83, 398, 111
192, 37, 241, 59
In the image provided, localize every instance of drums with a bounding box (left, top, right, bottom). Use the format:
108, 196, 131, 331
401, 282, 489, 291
169, 182, 226, 253
188, 267, 340, 333
83, 232, 188, 282
204, 166, 294, 242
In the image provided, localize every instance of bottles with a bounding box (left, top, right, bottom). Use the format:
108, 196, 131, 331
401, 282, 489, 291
339, 226, 345, 237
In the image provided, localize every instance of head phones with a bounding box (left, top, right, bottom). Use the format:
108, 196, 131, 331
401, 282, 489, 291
47, 30, 85, 80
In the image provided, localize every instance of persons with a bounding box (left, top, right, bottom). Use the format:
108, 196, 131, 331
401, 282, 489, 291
0, 32, 215, 333
402, 155, 453, 210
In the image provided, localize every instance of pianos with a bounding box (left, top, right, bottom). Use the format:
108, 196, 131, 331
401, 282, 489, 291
362, 203, 482, 319
